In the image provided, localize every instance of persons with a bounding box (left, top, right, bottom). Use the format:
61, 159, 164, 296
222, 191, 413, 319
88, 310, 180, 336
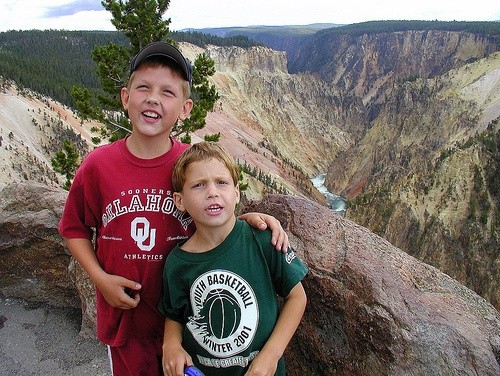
157, 140, 309, 376
60, 40, 290, 376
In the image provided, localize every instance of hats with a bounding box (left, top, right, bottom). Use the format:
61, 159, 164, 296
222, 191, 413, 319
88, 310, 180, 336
131, 41, 192, 84
